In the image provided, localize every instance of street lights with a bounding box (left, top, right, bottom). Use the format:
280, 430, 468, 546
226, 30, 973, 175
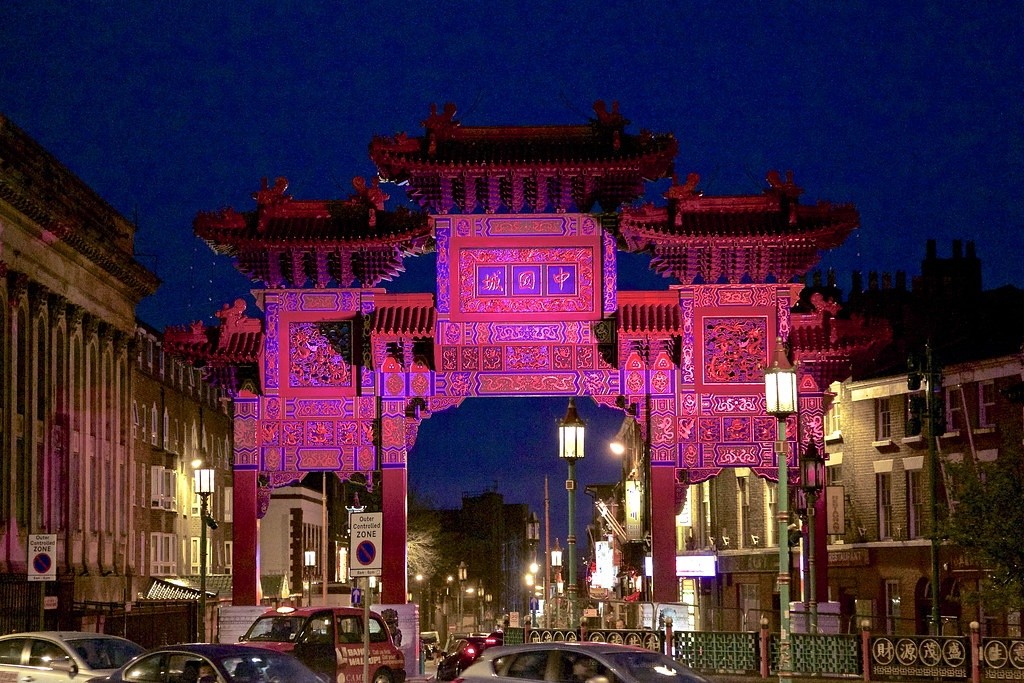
194, 448, 215, 644
796, 433, 825, 634
304, 538, 316, 607
759, 337, 799, 683
550, 537, 563, 630
525, 511, 540, 627
345, 492, 367, 609
558, 398, 584, 630
459, 588, 474, 632
555, 573, 565, 628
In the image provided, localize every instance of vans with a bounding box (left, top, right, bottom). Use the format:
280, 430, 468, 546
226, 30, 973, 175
235, 607, 406, 683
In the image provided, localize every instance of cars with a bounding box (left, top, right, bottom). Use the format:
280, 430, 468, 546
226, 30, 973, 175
420, 630, 503, 682
84, 642, 328, 682
0, 631, 151, 683
450, 640, 715, 683
